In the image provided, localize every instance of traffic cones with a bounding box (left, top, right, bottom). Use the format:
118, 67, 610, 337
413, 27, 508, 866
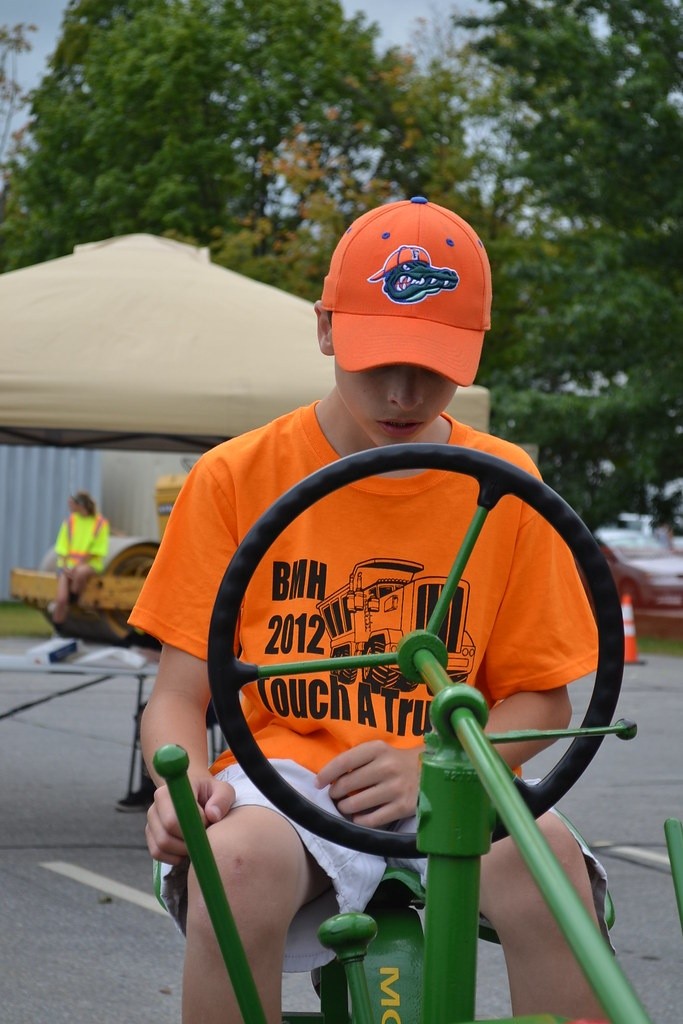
619, 595, 649, 667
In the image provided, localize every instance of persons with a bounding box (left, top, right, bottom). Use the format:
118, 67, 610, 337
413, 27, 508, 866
126, 198, 614, 1024
54, 490, 110, 626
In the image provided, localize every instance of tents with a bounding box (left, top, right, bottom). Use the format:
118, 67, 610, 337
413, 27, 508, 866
0, 233, 489, 456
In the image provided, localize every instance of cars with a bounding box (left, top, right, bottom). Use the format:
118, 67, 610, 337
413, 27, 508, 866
594, 514, 683, 608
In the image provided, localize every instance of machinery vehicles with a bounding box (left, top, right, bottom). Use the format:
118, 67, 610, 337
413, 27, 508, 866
10, 477, 187, 641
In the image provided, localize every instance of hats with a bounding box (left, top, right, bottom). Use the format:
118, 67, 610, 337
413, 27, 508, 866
320, 195, 492, 387
